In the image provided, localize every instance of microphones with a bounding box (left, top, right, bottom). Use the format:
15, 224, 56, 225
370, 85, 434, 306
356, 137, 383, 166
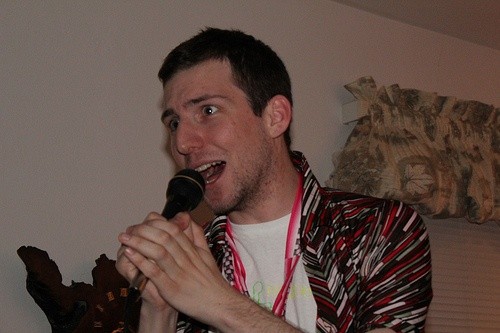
124, 168, 203, 309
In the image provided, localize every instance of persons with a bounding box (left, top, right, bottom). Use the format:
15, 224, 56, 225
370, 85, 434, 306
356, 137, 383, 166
115, 26, 434, 332
